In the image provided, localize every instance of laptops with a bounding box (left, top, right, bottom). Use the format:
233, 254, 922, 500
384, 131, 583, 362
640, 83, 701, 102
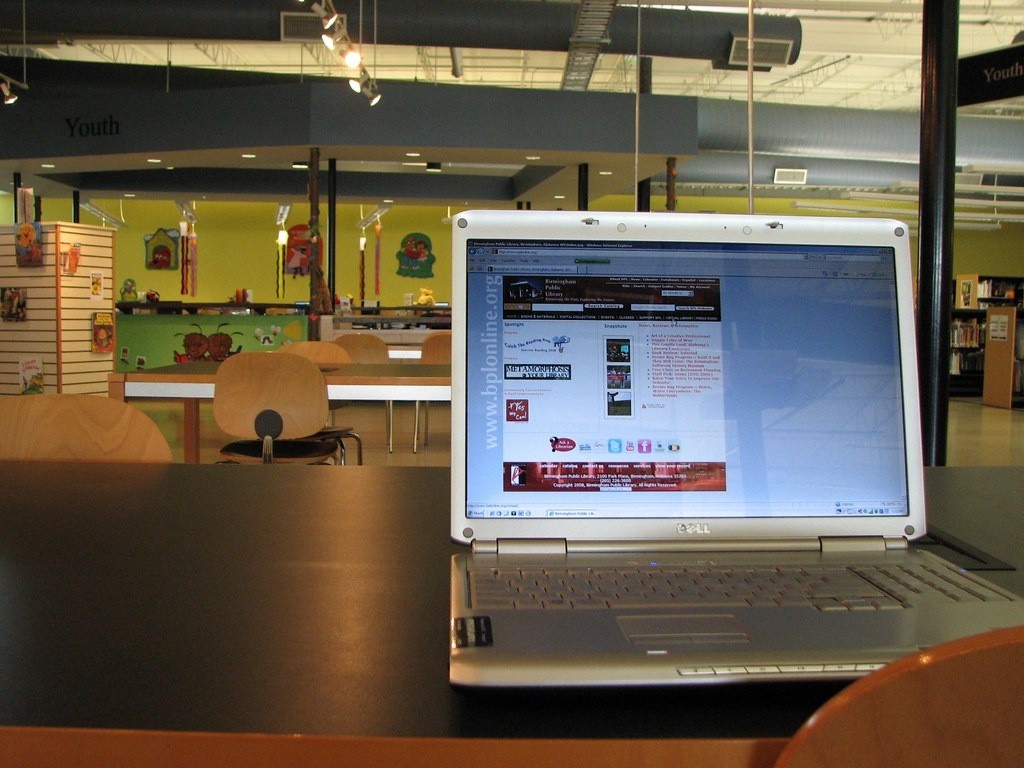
450, 210, 1024, 686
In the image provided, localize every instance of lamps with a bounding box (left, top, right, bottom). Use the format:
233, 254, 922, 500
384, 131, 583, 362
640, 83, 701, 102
0, 81, 18, 104
311, 1, 381, 106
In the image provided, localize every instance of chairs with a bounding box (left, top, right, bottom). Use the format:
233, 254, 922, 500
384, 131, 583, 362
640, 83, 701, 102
0, 335, 451, 465
775, 626, 1024, 767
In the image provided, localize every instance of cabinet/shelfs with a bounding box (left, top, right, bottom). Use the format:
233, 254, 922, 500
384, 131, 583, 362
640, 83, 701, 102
945, 274, 1024, 410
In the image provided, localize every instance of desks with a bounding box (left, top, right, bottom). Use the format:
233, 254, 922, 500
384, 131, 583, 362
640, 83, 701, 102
109, 361, 450, 463
0, 459, 1023, 767
333, 316, 451, 330
119, 302, 309, 315
353, 306, 451, 311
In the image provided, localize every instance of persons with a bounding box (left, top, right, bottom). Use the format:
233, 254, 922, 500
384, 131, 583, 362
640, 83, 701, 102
344, 294, 356, 314
414, 288, 434, 314
335, 292, 340, 304
611, 368, 625, 388
611, 346, 629, 360
8, 291, 24, 317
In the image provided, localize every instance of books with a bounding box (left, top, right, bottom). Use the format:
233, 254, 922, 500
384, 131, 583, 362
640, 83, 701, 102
952, 319, 987, 348
977, 281, 992, 309
958, 350, 983, 373
